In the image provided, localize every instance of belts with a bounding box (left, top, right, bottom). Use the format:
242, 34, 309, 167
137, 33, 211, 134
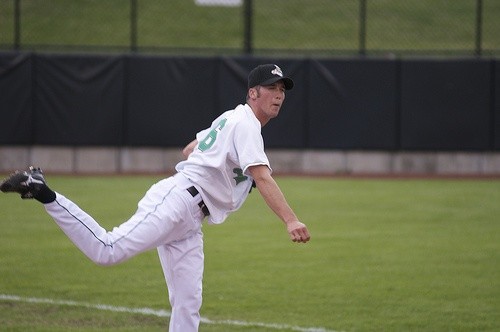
187, 186, 210, 217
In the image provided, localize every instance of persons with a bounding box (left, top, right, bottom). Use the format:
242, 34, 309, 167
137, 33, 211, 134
0, 64, 310, 332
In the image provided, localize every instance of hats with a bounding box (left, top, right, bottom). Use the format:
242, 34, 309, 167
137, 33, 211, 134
247, 63, 294, 90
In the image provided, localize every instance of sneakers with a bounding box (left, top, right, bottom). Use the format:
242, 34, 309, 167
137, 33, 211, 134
0, 166, 45, 200
